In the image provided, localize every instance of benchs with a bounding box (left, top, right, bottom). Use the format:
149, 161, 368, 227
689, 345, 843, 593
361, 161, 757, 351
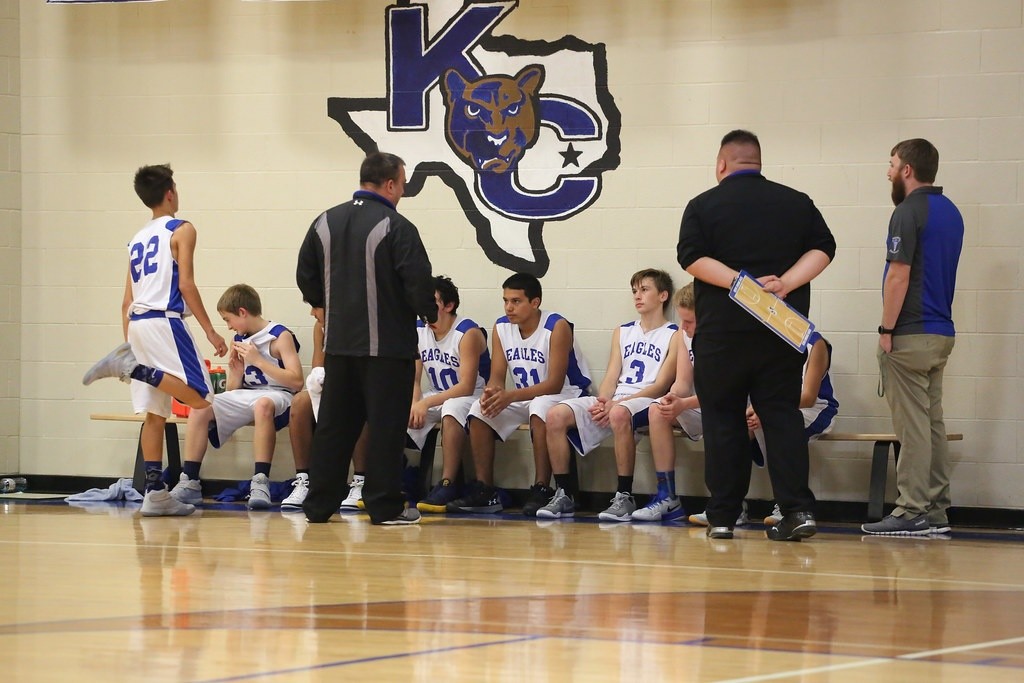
89, 415, 964, 523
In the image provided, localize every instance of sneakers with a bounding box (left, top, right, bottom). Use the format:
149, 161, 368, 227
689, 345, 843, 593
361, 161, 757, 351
140, 481, 196, 515
339, 511, 369, 529
246, 511, 271, 532
537, 487, 575, 517
522, 480, 555, 517
167, 472, 203, 505
280, 472, 309, 509
632, 493, 687, 522
188, 508, 203, 527
416, 478, 472, 513
633, 525, 683, 545
534, 519, 574, 533
600, 523, 631, 539
82, 344, 139, 386
248, 473, 272, 507
142, 517, 188, 541
598, 491, 637, 522
339, 478, 366, 509
281, 510, 309, 533
375, 507, 421, 524
446, 480, 503, 514
689, 499, 954, 567
383, 524, 421, 541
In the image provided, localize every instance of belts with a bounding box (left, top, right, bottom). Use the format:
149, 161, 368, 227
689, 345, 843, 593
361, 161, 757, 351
131, 311, 181, 318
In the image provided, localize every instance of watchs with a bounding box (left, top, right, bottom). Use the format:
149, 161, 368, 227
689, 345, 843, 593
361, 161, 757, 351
878, 325, 894, 334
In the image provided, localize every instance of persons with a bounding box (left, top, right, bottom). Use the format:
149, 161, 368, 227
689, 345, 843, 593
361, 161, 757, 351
690, 330, 839, 524
861, 136, 964, 537
632, 281, 749, 525
168, 282, 304, 514
676, 131, 837, 543
533, 268, 680, 522
81, 167, 226, 517
282, 291, 369, 510
295, 152, 441, 525
445, 272, 593, 516
357, 276, 492, 514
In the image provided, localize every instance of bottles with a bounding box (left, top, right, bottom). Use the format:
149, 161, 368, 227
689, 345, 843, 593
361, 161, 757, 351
213, 365, 227, 394
207, 366, 215, 394
